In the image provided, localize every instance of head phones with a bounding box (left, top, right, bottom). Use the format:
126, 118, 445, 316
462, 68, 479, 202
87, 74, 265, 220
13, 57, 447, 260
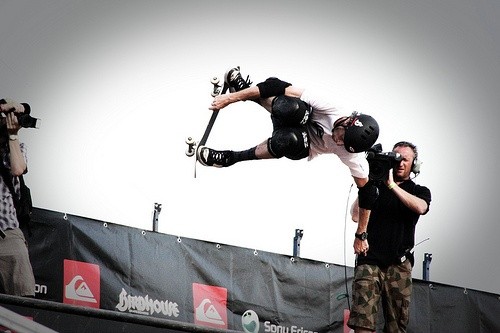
411, 161, 421, 174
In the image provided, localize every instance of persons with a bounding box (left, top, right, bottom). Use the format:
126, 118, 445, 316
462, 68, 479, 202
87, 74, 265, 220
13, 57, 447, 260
0, 99, 36, 298
196, 65, 380, 256
346, 141, 431, 333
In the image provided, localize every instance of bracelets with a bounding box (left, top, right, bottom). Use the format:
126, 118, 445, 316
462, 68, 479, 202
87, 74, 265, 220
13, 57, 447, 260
388, 181, 396, 190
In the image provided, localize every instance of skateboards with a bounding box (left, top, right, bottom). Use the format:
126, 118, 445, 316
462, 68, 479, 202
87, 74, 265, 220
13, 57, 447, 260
185, 66, 242, 179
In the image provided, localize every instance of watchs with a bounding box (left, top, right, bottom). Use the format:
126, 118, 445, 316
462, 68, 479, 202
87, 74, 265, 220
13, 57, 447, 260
355, 232, 368, 241
9, 135, 18, 141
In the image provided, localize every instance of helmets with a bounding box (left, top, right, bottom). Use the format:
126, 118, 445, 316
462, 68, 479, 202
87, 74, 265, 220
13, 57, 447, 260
343, 113, 380, 153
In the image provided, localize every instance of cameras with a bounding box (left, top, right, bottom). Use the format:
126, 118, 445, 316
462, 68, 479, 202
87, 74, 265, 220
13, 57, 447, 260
16, 113, 42, 129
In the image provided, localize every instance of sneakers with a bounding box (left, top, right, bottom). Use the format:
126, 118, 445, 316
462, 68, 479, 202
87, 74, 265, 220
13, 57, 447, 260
197, 146, 232, 170
225, 66, 251, 99
